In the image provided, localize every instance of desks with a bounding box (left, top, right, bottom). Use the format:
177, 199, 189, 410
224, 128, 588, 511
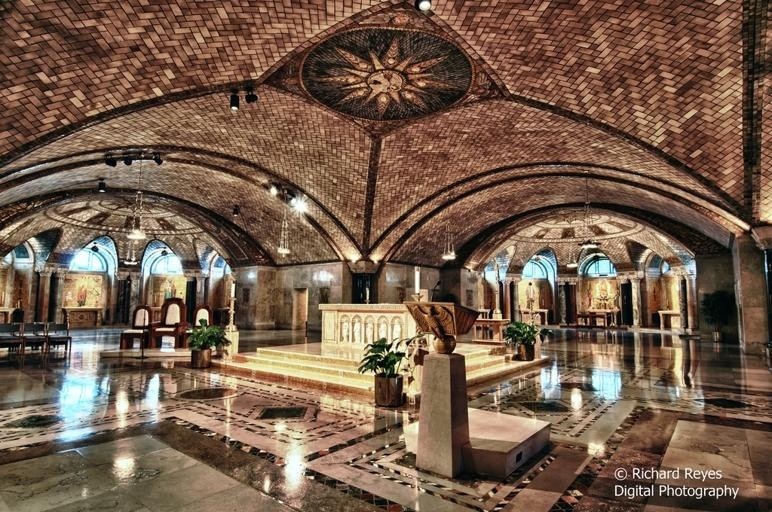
588, 308, 619, 328
61, 307, 104, 330
658, 310, 681, 330
520, 308, 549, 326
473, 319, 510, 341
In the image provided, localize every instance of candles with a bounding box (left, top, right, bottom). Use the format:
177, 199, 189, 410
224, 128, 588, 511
414, 266, 421, 294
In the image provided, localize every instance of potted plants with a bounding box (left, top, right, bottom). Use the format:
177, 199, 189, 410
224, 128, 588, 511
184, 318, 232, 369
700, 291, 736, 342
359, 337, 414, 408
503, 320, 544, 361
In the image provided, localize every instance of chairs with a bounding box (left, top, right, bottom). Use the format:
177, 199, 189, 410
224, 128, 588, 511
0, 321, 72, 367
478, 309, 491, 340
120, 297, 214, 349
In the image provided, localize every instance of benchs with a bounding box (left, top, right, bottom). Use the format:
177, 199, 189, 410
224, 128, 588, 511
576, 314, 605, 328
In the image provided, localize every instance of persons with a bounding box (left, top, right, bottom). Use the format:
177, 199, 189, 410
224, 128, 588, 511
488, 302, 497, 320
526, 281, 536, 300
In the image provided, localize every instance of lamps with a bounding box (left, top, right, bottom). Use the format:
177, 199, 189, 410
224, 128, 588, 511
565, 235, 578, 270
229, 88, 239, 113
414, 0, 432, 13
441, 220, 456, 261
577, 176, 597, 248
243, 80, 258, 104
90, 147, 170, 258
278, 189, 291, 258
233, 204, 240, 217
